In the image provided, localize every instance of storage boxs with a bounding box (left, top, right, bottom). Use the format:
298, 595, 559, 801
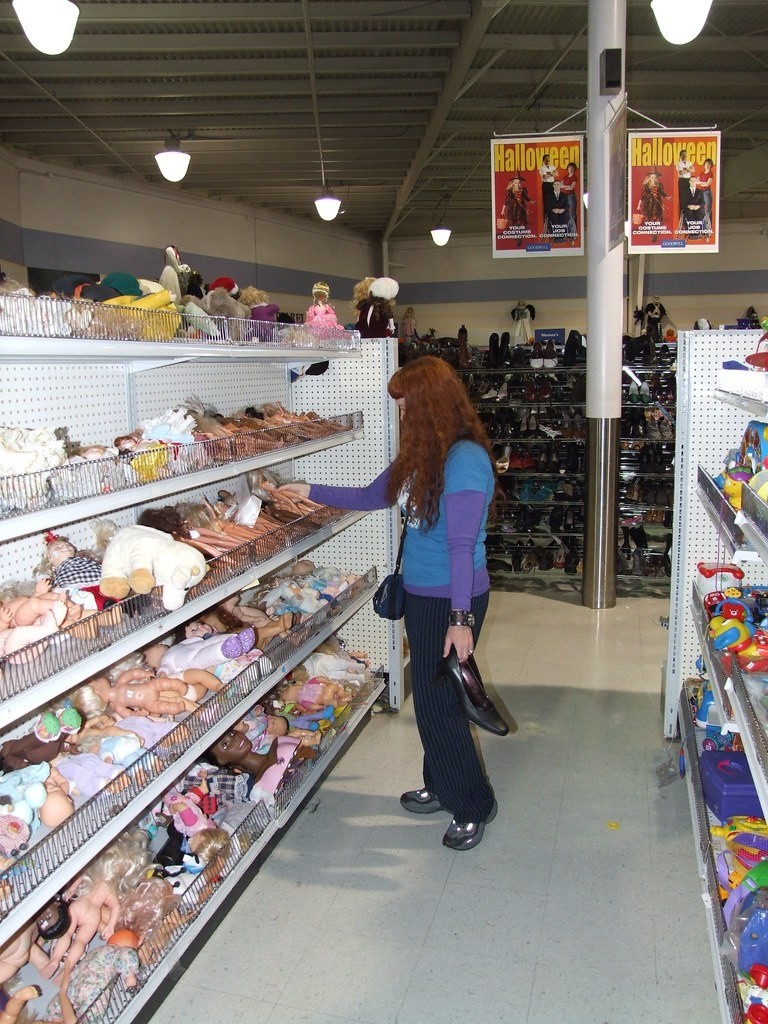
696, 750, 765, 823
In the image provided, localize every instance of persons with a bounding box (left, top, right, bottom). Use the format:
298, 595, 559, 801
675, 150, 716, 242
514, 299, 531, 344
0, 401, 365, 1024
268, 357, 500, 851
401, 307, 421, 341
637, 167, 673, 242
305, 282, 353, 340
501, 175, 537, 247
540, 154, 578, 247
646, 295, 671, 343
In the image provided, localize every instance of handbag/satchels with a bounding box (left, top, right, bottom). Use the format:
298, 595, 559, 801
373, 574, 406, 620
633, 210, 644, 226
497, 215, 508, 229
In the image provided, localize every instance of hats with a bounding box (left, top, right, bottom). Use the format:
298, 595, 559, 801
509, 171, 525, 181
647, 161, 662, 176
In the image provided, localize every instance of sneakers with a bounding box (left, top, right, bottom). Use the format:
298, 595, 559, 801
442, 798, 498, 850
400, 787, 446, 814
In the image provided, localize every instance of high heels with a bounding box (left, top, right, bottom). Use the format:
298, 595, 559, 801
399, 326, 678, 576
431, 650, 509, 736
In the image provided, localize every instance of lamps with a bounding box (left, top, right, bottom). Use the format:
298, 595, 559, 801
313, 190, 342, 222
12, 0, 82, 56
153, 134, 192, 183
650, 0, 714, 46
430, 227, 451, 246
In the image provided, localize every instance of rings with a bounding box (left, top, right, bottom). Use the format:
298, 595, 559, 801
469, 649, 474, 653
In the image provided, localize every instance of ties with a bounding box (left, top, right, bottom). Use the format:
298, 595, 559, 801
692, 189, 694, 195
557, 193, 559, 200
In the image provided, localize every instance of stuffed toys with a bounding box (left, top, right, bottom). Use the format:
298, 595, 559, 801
100, 524, 210, 611
355, 276, 399, 339
0, 246, 280, 343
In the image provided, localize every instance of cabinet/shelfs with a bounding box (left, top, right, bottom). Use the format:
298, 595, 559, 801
452, 341, 677, 582
0, 291, 404, 1024
661, 327, 768, 1024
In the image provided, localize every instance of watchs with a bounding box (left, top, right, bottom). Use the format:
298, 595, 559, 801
448, 610, 475, 628
